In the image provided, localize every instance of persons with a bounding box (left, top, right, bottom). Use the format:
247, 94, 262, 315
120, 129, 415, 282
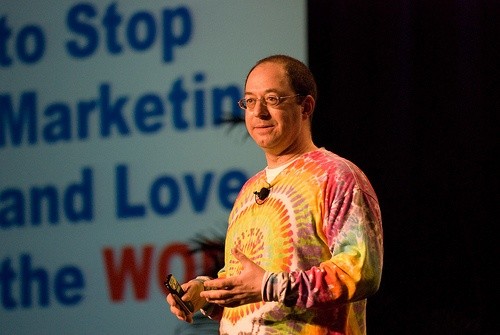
167, 55, 383, 335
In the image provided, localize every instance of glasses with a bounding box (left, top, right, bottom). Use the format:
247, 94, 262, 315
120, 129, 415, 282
238, 94, 307, 111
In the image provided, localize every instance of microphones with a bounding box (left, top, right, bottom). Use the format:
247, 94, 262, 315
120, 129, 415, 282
253, 187, 270, 200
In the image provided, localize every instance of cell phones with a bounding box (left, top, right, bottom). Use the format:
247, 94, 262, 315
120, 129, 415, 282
164, 274, 194, 315
